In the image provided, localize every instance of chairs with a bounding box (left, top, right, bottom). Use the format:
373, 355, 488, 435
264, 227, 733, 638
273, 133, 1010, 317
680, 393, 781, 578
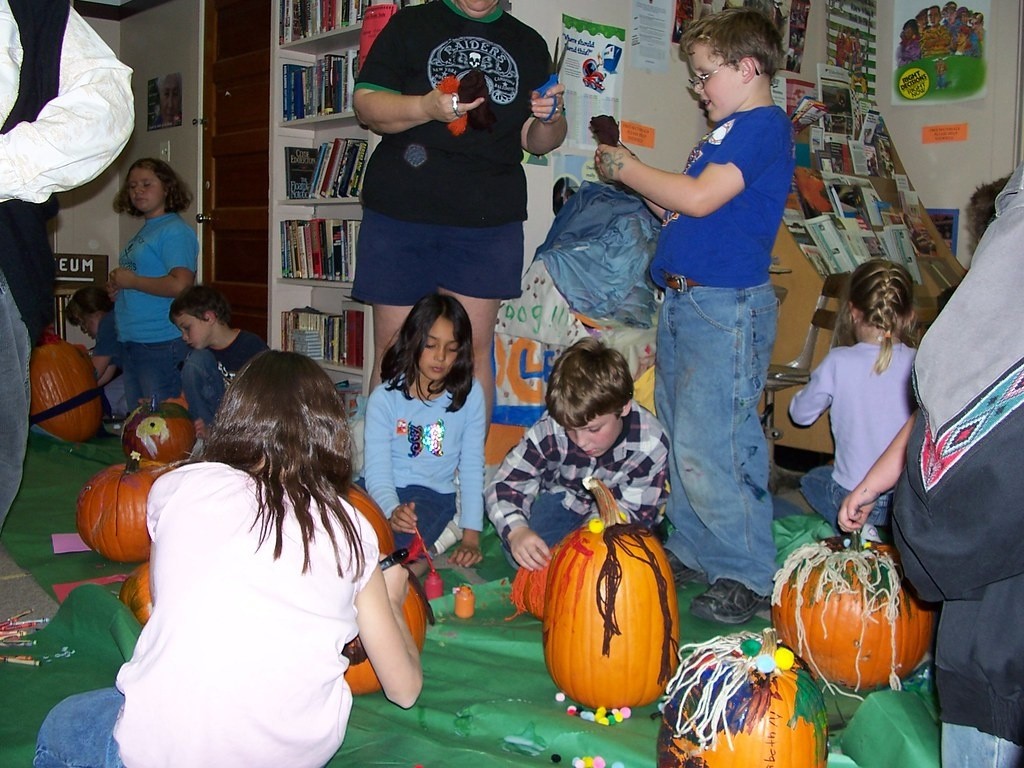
762, 270, 856, 492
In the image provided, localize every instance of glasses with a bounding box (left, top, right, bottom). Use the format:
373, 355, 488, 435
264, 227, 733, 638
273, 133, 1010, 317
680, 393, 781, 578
688, 57, 760, 90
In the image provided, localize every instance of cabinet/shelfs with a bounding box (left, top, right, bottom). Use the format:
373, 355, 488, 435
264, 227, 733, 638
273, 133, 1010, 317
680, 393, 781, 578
268, 0, 419, 399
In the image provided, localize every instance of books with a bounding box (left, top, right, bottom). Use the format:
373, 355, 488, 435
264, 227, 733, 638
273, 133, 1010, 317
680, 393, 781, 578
772, 70, 961, 301
278, 0, 430, 414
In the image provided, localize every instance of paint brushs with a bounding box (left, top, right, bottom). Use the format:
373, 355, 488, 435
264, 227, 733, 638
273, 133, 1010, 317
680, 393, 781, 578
413, 523, 438, 576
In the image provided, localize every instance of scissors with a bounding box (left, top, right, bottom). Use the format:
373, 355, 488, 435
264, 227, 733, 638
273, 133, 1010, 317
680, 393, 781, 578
529, 36, 569, 122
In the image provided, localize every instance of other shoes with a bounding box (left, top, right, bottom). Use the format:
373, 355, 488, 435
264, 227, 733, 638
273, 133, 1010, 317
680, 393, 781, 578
433, 521, 463, 553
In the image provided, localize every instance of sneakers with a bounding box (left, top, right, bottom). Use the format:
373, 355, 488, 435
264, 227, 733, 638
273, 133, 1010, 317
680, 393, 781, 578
663, 546, 699, 586
691, 577, 769, 624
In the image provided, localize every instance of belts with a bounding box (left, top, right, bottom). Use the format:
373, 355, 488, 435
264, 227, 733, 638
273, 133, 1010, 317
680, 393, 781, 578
662, 272, 695, 293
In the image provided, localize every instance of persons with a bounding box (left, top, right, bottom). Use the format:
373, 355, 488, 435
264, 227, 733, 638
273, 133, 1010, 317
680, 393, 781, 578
594, 6, 794, 626
104, 157, 199, 411
63, 286, 130, 422
0, 0, 136, 529
151, 72, 182, 128
167, 285, 269, 444
932, 174, 1012, 318
354, 293, 486, 577
787, 260, 919, 538
839, 164, 1024, 768
348, 0, 567, 446
481, 336, 671, 571
30, 350, 424, 768
